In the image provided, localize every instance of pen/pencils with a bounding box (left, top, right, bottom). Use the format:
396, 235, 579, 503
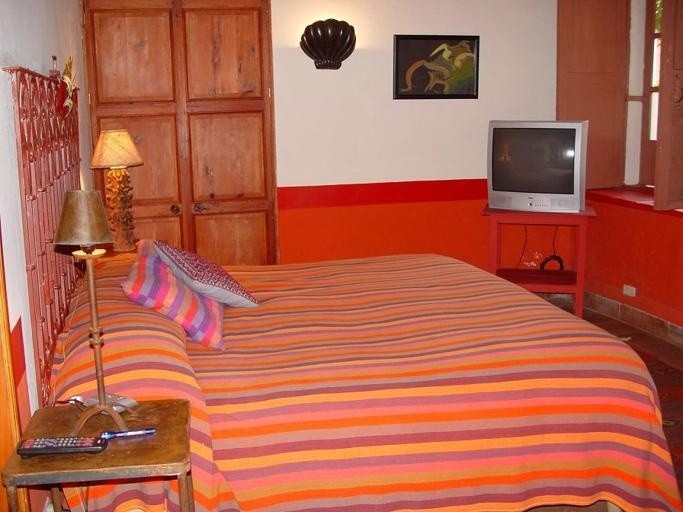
101, 428, 157, 439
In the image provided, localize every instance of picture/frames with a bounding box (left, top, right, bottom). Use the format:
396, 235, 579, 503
393, 34, 479, 99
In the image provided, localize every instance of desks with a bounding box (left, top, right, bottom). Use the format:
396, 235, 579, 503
481, 203, 596, 319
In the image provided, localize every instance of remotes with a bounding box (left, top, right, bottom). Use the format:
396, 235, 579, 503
18, 434, 107, 457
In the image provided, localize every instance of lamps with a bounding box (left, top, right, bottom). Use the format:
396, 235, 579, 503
52, 187, 137, 439
89, 129, 144, 253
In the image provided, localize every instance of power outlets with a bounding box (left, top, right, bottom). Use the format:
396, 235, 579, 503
623, 284, 636, 297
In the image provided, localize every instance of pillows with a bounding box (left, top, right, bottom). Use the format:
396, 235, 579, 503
42, 254, 242, 511
154, 238, 259, 307
122, 238, 225, 352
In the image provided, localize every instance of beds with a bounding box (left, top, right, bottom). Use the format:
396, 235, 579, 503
39, 254, 683, 512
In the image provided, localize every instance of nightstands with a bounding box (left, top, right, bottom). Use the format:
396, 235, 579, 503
0, 400, 194, 511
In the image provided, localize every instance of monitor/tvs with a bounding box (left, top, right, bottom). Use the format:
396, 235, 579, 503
486, 119, 589, 213
